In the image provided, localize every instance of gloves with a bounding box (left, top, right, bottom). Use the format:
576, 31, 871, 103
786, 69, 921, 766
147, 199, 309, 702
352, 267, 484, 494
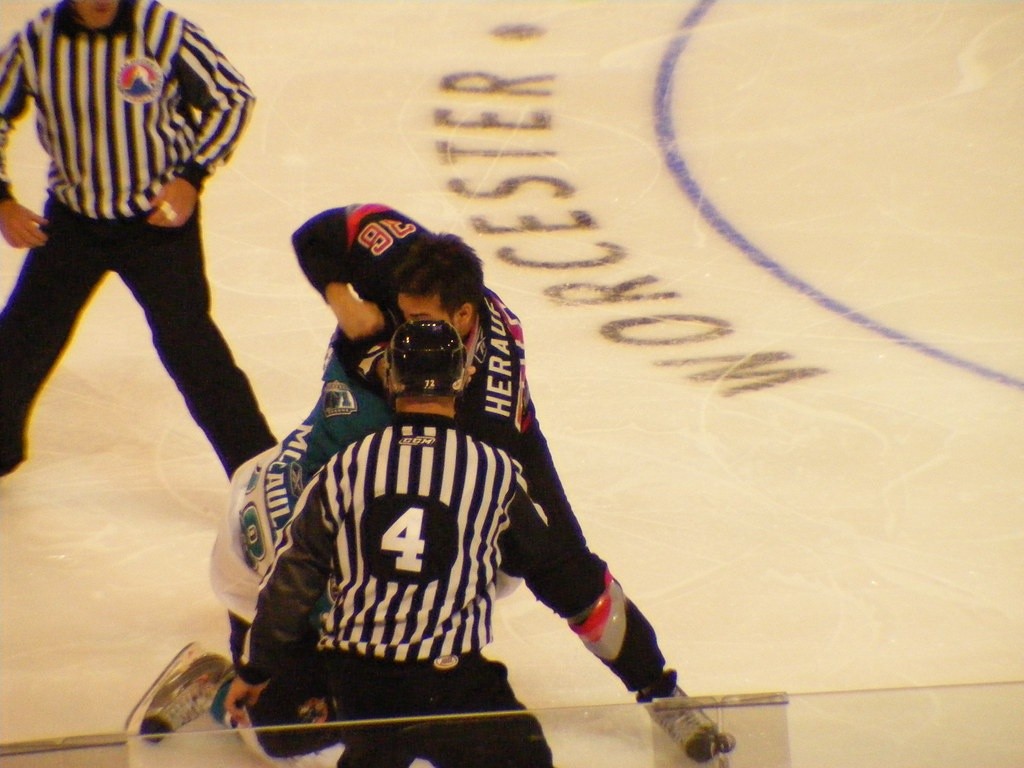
246, 670, 339, 759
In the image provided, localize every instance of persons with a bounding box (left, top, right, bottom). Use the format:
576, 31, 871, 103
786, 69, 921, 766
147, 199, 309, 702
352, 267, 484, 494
0, 0, 280, 482
140, 203, 736, 768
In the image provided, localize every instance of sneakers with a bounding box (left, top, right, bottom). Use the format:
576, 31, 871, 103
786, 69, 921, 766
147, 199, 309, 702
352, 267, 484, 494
125, 641, 237, 746
636, 670, 735, 768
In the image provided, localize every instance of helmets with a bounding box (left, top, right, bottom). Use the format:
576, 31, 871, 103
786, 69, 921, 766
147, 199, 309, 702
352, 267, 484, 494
387, 319, 468, 396
